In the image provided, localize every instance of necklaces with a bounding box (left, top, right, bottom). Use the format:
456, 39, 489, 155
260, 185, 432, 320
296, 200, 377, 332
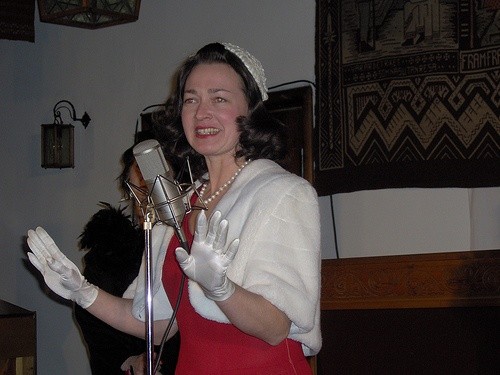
199, 159, 253, 204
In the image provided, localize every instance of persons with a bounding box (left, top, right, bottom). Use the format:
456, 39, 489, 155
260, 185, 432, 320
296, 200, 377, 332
73, 130, 198, 375
27, 42, 323, 375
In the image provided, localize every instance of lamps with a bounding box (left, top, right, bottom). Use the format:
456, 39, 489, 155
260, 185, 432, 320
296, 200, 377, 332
38, 0, 141, 30
41, 100, 91, 170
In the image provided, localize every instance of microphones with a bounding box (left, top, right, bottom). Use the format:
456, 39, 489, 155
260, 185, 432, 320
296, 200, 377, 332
133, 139, 190, 255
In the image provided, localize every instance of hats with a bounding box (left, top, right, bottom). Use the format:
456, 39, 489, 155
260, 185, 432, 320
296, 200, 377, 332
220, 42, 269, 102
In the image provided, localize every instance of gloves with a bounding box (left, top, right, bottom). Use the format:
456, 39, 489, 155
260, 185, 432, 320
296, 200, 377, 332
175, 210, 240, 302
27, 226, 99, 309
